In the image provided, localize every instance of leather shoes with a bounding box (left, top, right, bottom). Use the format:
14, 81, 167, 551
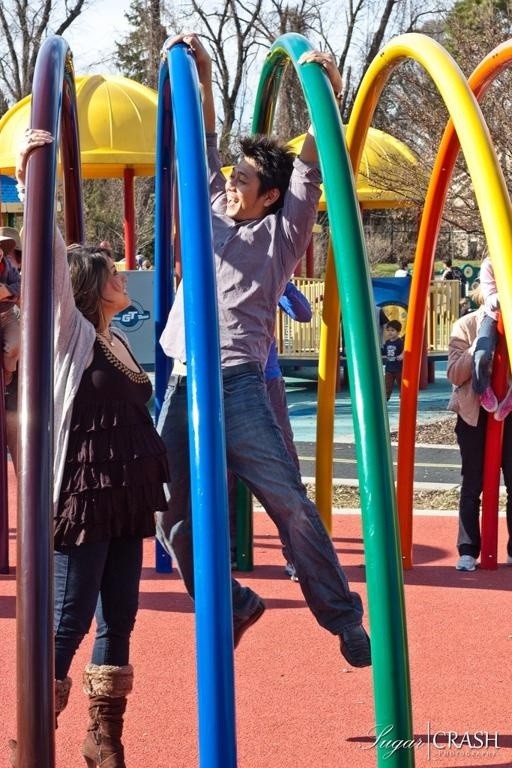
233, 601, 265, 651
340, 623, 371, 668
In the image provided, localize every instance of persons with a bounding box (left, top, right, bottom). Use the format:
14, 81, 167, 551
380, 320, 405, 402
264, 281, 314, 587
0, 127, 139, 768
446, 252, 512, 572
156, 32, 372, 668
394, 258, 468, 298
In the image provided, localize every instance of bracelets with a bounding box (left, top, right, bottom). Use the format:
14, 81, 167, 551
334, 90, 342, 100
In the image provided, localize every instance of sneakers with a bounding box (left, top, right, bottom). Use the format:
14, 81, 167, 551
479, 385, 512, 422
455, 555, 476, 571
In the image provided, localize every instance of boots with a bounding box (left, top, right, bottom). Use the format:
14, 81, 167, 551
55, 677, 73, 730
83, 664, 133, 768
